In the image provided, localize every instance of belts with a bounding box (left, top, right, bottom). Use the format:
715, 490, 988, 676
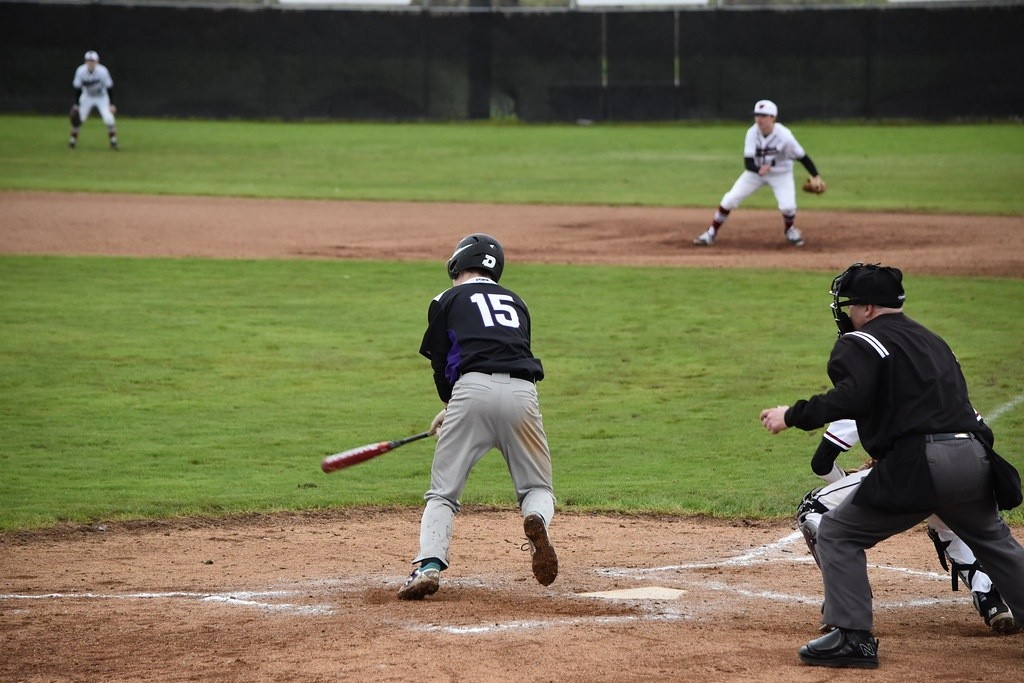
926, 432, 975, 442
461, 369, 538, 385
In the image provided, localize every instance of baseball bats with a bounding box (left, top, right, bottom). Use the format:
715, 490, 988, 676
320, 430, 434, 474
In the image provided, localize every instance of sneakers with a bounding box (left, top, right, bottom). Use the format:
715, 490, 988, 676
398, 562, 440, 600
68, 132, 78, 146
108, 131, 117, 145
693, 230, 715, 245
799, 628, 880, 669
522, 509, 558, 587
973, 583, 1013, 631
784, 225, 804, 248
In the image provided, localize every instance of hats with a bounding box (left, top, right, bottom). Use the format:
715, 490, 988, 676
754, 99, 778, 117
84, 51, 99, 62
842, 263, 906, 309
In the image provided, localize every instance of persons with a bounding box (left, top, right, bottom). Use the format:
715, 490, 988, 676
759, 261, 1024, 669
67, 50, 121, 152
396, 232, 558, 600
795, 406, 1016, 634
692, 99, 826, 248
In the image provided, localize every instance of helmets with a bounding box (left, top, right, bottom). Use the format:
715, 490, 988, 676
447, 233, 505, 283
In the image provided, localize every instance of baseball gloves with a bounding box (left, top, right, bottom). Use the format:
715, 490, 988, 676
847, 459, 877, 475
69, 108, 81, 127
802, 175, 825, 194
